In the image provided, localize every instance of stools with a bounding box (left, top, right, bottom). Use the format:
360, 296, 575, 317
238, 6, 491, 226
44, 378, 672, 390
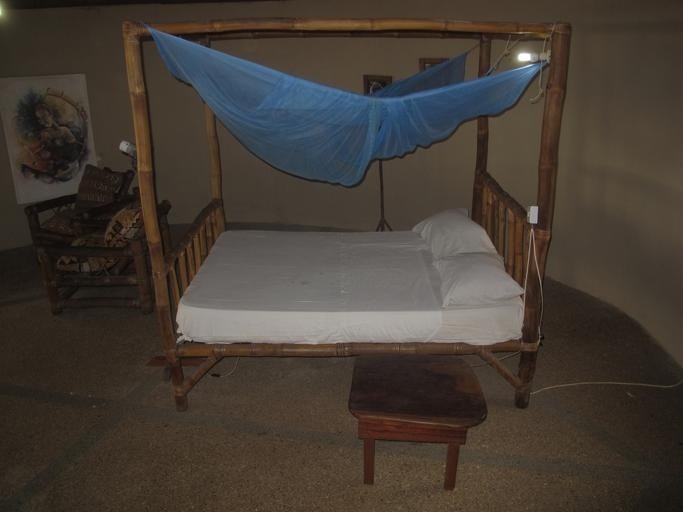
348, 355, 487, 489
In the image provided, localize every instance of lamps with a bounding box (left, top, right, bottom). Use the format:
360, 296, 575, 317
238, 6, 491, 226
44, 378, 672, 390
517, 52, 552, 63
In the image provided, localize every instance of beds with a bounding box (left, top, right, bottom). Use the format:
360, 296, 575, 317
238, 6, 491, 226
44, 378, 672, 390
119, 19, 571, 412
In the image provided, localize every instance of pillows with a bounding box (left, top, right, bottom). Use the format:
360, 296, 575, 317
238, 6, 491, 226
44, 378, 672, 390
412, 207, 497, 256
430, 251, 524, 307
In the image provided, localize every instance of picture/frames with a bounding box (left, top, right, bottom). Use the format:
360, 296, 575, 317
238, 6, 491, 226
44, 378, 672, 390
360, 75, 391, 92
418, 55, 446, 77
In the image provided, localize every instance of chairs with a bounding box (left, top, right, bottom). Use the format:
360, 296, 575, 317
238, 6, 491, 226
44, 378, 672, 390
24, 164, 172, 314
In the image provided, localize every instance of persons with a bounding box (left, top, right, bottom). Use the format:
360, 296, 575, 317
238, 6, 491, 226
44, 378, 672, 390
34, 103, 76, 147
